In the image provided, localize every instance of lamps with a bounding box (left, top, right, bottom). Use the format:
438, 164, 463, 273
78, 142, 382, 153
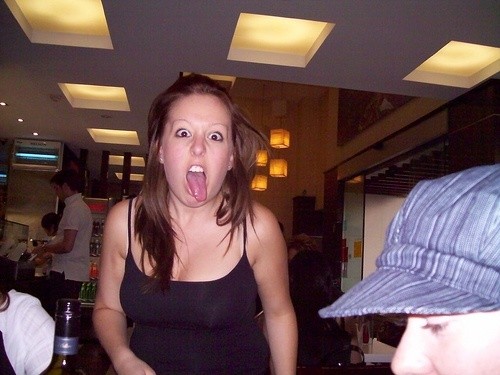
251, 79, 290, 192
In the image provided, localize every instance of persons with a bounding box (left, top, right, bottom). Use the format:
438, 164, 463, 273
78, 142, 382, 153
31, 168, 94, 312
41, 212, 63, 240
91, 73, 299, 375
317, 164, 500, 375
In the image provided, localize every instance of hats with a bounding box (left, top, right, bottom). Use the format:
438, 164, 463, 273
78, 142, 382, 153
318, 164, 500, 318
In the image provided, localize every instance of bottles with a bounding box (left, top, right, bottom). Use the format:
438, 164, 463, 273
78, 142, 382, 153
81, 283, 97, 303
90, 239, 103, 257
91, 218, 104, 237
90, 260, 97, 277
39, 299, 89, 375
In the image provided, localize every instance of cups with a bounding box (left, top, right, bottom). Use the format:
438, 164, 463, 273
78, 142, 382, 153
344, 316, 369, 346
